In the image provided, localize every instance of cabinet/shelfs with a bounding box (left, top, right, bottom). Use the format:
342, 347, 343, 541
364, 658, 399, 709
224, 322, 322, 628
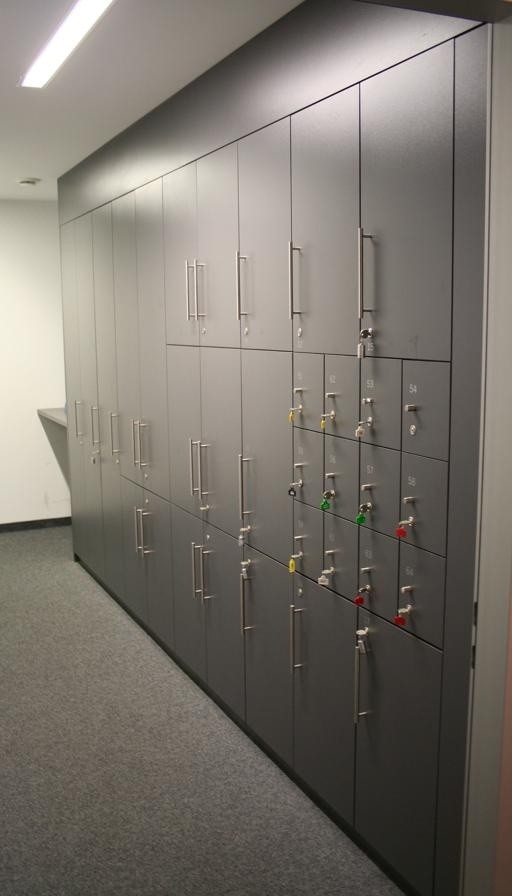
58, 0, 512, 896
